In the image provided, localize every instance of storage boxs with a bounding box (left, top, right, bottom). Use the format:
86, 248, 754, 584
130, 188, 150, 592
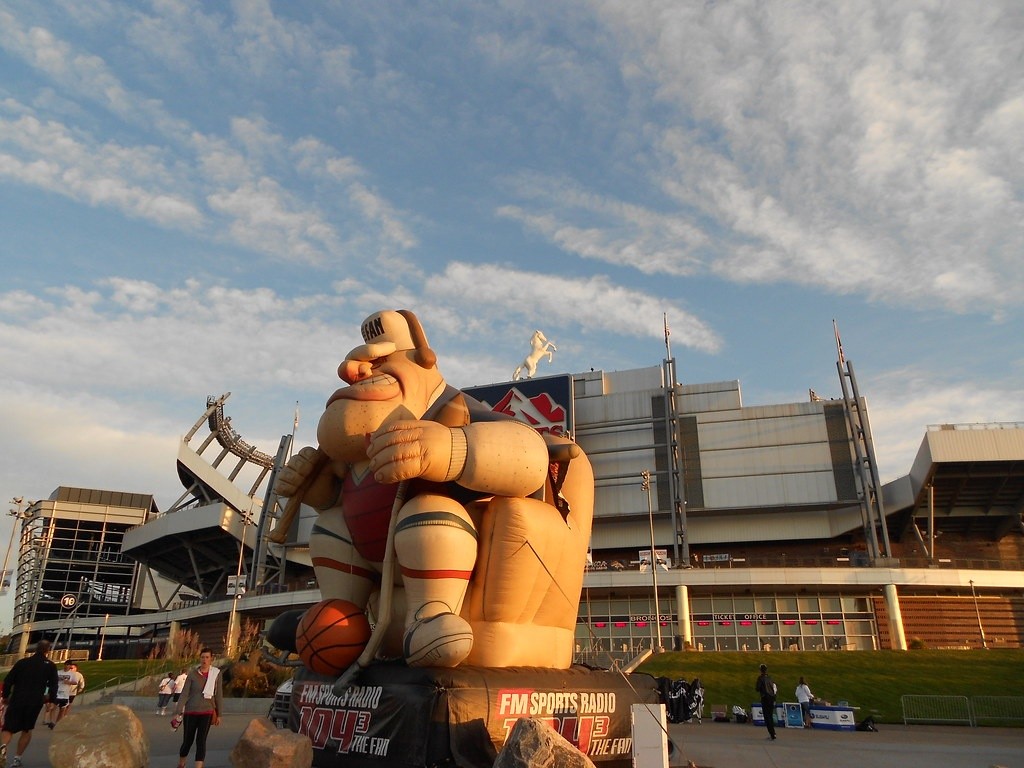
736, 712, 747, 723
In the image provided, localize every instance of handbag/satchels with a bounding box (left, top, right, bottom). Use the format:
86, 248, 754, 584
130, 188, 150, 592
211, 709, 217, 725
160, 686, 165, 691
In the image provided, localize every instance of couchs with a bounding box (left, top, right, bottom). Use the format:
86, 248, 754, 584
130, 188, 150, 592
367, 429, 594, 672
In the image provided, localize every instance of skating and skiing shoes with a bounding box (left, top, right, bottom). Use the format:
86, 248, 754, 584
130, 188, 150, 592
768, 733, 777, 744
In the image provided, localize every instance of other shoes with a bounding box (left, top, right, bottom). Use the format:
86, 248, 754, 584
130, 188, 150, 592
161, 712, 166, 715
804, 724, 814, 729
0, 745, 7, 768
156, 710, 159, 714
11, 758, 24, 768
177, 762, 185, 768
42, 721, 50, 725
48, 722, 55, 730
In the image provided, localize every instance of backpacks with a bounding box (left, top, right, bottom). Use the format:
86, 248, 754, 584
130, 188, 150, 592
760, 677, 777, 698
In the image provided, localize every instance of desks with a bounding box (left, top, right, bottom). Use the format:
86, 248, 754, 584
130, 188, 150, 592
829, 705, 861, 730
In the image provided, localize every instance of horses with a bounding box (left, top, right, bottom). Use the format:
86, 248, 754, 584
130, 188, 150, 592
512, 330, 556, 380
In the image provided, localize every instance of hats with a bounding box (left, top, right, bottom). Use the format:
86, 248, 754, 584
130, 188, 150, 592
64, 660, 72, 666
759, 664, 767, 670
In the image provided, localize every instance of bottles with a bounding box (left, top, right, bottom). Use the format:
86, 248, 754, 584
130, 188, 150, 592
170, 715, 182, 732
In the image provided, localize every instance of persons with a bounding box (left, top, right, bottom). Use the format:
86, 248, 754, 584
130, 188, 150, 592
795, 676, 814, 727
0, 639, 59, 768
755, 664, 776, 741
155, 669, 188, 715
171, 648, 224, 767
43, 659, 85, 730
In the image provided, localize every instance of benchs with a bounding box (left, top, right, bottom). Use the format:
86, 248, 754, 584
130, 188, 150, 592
711, 703, 727, 721
